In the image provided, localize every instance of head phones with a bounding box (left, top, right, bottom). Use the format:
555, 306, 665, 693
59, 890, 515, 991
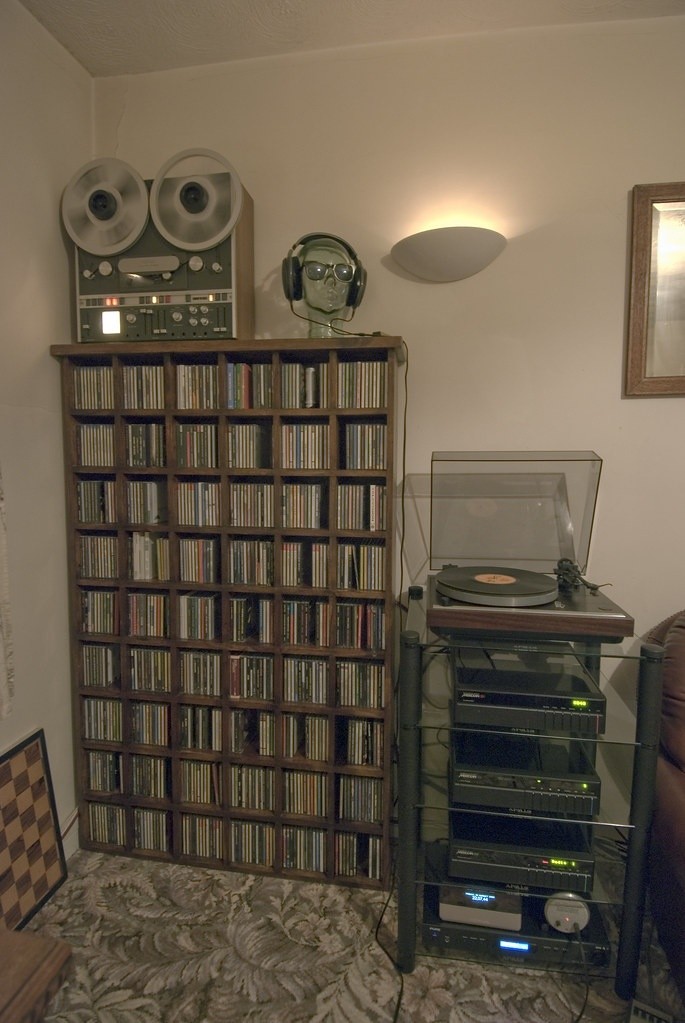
282, 232, 367, 308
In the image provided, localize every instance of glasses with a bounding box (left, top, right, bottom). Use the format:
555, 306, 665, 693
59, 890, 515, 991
302, 260, 357, 282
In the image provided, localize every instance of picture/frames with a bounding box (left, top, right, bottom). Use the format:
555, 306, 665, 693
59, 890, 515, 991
0, 727, 68, 932
624, 181, 685, 396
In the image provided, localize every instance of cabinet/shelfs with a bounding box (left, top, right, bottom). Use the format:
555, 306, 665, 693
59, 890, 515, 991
397, 585, 665, 1000
50, 336, 405, 890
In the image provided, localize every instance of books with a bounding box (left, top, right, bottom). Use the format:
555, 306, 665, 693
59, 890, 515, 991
74, 362, 388, 882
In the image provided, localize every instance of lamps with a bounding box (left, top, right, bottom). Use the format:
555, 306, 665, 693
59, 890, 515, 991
391, 227, 506, 281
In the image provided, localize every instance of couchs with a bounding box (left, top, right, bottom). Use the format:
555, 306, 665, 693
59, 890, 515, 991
647, 610, 685, 1007
